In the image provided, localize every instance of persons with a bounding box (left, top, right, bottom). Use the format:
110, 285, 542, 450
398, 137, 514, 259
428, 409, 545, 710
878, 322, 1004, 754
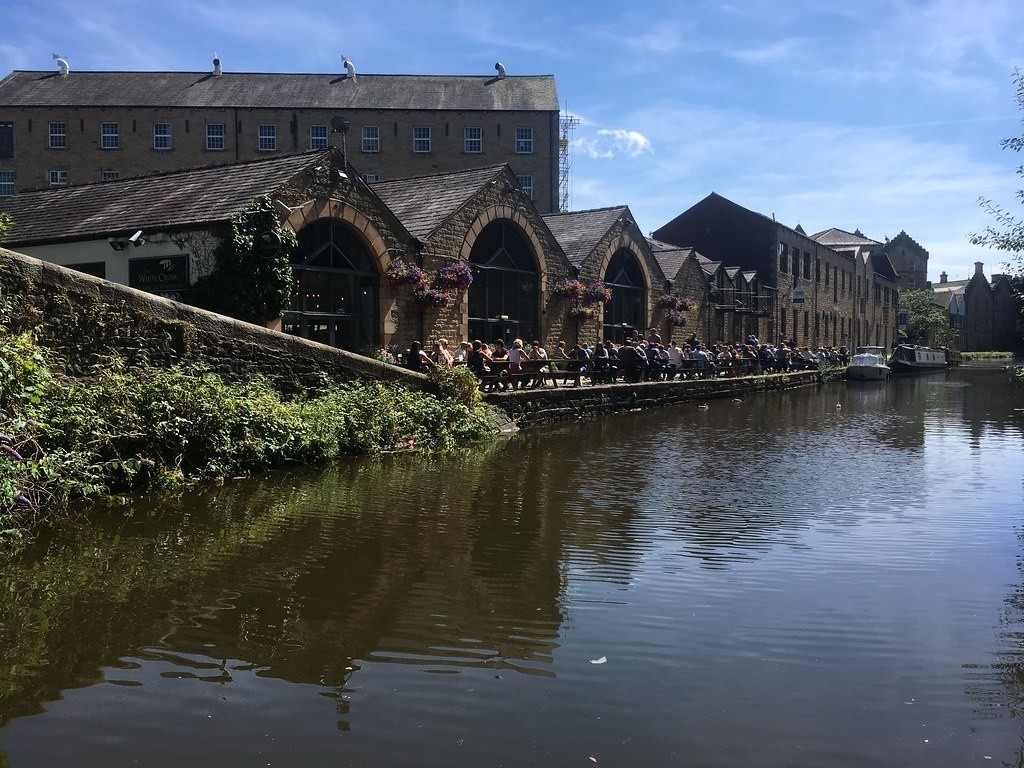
403, 328, 851, 393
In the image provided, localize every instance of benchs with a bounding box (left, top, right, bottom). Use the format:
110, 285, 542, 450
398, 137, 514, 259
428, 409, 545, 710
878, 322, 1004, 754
467, 375, 498, 393
550, 371, 567, 385
679, 368, 709, 372
570, 371, 580, 387
520, 373, 548, 389
507, 374, 523, 390
586, 370, 602, 385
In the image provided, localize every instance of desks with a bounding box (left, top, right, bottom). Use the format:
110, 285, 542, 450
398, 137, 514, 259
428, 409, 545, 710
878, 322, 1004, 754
682, 359, 703, 380
568, 358, 595, 364
521, 358, 565, 363
452, 360, 516, 366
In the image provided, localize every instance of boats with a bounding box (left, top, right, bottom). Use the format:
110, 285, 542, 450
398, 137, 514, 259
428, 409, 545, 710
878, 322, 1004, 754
847, 353, 893, 383
887, 345, 949, 372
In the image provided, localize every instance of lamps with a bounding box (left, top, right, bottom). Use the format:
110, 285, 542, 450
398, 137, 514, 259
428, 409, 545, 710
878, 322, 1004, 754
618, 217, 633, 229
501, 180, 517, 196
275, 200, 304, 213
109, 240, 129, 251
333, 167, 347, 182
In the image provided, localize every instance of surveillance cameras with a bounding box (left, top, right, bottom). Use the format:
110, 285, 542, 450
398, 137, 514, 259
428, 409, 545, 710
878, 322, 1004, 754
129, 231, 142, 242
276, 200, 292, 214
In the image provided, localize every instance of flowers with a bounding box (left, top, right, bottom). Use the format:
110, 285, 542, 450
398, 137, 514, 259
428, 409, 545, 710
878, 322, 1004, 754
554, 276, 586, 299
387, 259, 432, 289
655, 294, 678, 310
435, 264, 472, 289
570, 302, 599, 320
415, 287, 454, 311
678, 298, 698, 312
586, 283, 614, 301
666, 310, 688, 327
